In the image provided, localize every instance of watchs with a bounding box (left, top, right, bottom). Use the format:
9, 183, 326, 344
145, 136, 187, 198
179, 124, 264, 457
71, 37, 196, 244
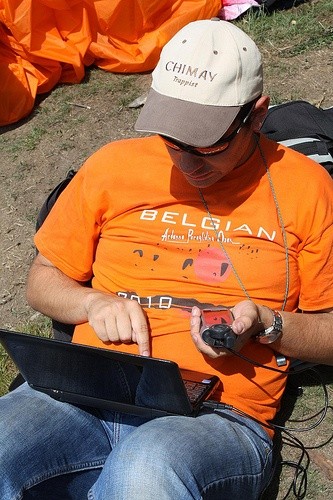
251, 309, 282, 345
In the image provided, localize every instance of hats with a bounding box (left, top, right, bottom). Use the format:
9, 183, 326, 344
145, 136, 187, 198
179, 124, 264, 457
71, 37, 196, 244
134, 17, 263, 150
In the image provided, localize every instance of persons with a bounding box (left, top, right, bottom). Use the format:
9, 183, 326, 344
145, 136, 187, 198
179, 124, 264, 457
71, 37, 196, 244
0, 18, 333, 500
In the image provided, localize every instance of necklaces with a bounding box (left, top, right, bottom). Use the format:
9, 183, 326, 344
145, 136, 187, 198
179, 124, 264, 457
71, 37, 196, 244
198, 133, 289, 367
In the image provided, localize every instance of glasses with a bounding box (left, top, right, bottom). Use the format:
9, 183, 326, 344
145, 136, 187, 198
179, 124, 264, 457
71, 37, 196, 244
157, 102, 257, 157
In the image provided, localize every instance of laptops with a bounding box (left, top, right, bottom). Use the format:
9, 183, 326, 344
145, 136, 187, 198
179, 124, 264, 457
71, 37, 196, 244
0, 329, 220, 419
